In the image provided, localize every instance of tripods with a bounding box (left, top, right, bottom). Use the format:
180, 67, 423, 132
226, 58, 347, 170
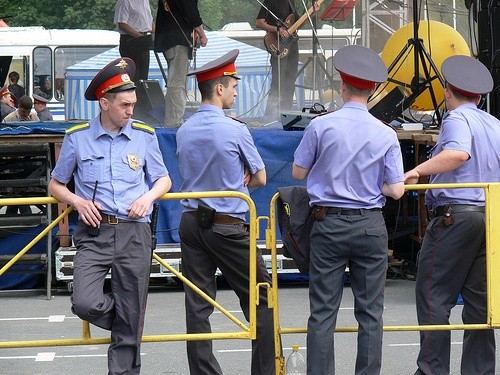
293, 0, 343, 107
366, 0, 446, 125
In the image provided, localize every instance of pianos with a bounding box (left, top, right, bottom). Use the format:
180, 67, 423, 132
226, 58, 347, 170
123, 33, 168, 88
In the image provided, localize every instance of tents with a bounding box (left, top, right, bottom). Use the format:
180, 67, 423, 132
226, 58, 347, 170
64, 30, 305, 120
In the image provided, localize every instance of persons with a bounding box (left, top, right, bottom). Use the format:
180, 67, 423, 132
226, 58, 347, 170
113, 0, 156, 117
405, 54, 499, 375
154, 0, 208, 129
176, 49, 284, 375
0, 71, 53, 123
48, 58, 172, 375
292, 45, 405, 375
256, 0, 320, 126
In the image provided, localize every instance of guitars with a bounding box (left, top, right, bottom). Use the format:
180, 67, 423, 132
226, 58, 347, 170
187, 29, 201, 60
264, 0, 324, 59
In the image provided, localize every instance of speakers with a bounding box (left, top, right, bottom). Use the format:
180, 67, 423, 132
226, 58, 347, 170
478, 0, 500, 121
129, 79, 165, 128
368, 86, 417, 125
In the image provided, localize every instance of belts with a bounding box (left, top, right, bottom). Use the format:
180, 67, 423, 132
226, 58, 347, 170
99, 210, 127, 223
139, 32, 152, 36
323, 206, 377, 217
188, 208, 243, 225
432, 200, 486, 214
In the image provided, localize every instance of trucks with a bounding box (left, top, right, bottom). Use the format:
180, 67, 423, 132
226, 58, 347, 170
0, 26, 120, 120
213, 22, 362, 110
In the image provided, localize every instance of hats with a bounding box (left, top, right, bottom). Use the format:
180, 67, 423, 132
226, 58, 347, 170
441, 54, 493, 95
0, 83, 13, 95
33, 77, 40, 83
187, 49, 243, 81
334, 44, 389, 89
85, 57, 135, 100
32, 92, 49, 104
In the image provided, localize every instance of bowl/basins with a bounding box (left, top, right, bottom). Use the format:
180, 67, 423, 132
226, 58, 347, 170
401, 123, 424, 130
